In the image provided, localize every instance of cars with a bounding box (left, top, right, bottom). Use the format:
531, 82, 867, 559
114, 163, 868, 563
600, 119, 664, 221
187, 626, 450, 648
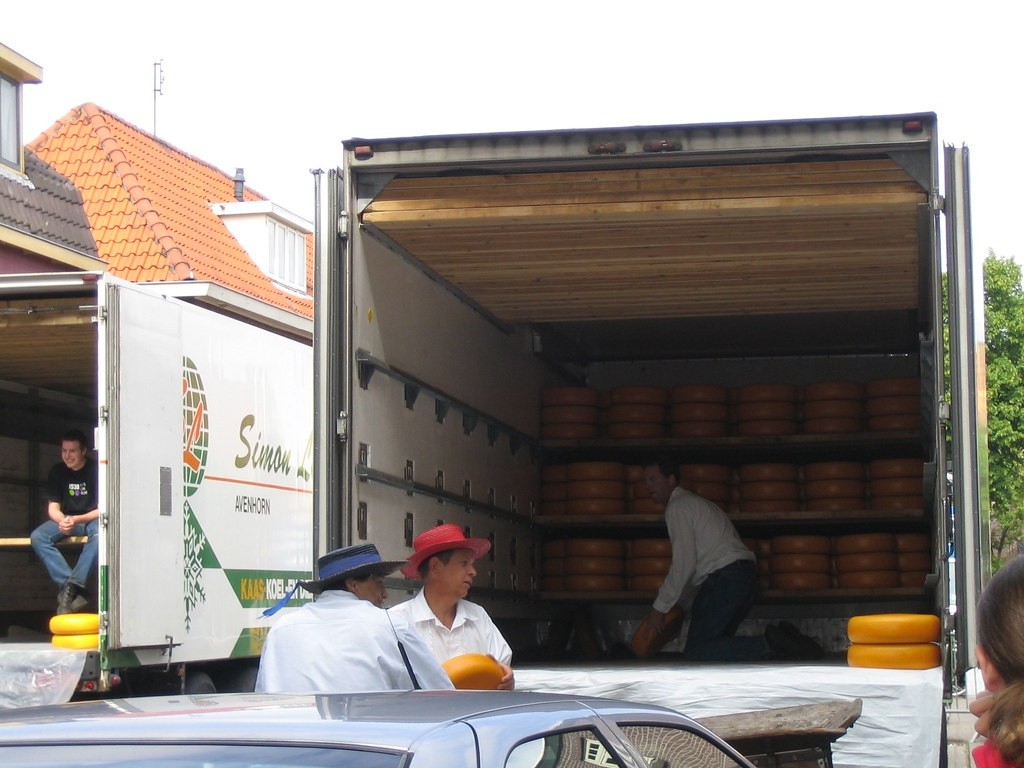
0, 687, 759, 768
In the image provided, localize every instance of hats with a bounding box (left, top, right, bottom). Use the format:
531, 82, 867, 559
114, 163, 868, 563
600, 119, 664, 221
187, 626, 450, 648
299, 544, 409, 594
400, 524, 491, 578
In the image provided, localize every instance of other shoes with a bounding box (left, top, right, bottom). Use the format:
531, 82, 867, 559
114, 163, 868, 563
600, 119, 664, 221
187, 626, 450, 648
765, 620, 821, 660
57, 582, 78, 613
70, 589, 95, 611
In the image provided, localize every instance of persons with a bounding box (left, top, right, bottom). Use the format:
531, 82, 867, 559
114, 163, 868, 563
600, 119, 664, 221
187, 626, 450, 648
30, 428, 98, 615
253, 543, 456, 693
640, 461, 822, 661
386, 524, 515, 690
969, 553, 1024, 768
